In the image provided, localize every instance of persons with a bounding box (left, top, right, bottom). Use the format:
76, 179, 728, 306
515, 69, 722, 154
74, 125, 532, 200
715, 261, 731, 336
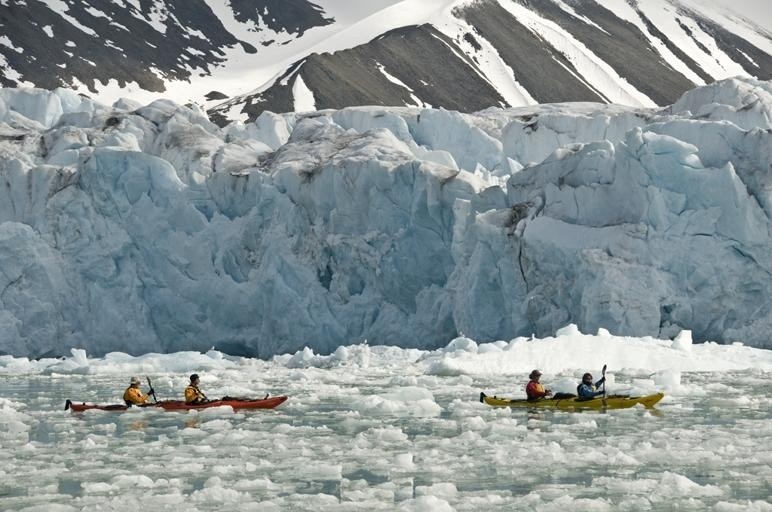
576, 373, 607, 398
122, 376, 155, 405
526, 370, 552, 400
184, 374, 208, 402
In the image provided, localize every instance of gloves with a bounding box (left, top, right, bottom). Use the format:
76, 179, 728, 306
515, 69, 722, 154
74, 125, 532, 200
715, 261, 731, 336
64, 394, 289, 412
480, 390, 664, 411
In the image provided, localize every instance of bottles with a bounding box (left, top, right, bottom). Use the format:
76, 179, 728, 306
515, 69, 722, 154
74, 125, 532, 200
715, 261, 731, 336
190, 374, 198, 381
532, 370, 543, 376
130, 376, 141, 385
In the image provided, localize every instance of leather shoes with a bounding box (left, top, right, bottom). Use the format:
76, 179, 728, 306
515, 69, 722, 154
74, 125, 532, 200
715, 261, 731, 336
148, 389, 154, 394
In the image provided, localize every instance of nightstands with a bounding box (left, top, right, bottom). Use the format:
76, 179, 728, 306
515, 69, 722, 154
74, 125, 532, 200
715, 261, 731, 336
602, 364, 607, 399
